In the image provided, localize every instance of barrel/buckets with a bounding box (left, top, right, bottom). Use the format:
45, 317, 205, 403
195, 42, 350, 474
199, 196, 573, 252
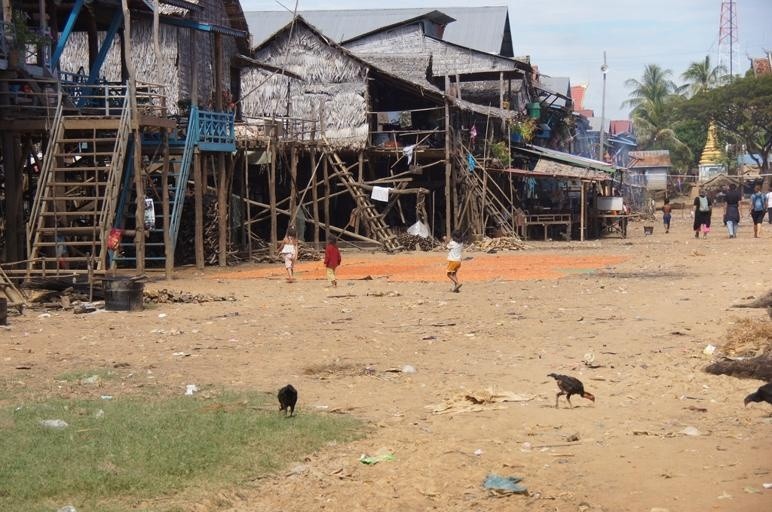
643, 219, 654, 234
103, 274, 148, 311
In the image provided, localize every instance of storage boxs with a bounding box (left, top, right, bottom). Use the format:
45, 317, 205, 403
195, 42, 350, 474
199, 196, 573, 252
596, 196, 623, 211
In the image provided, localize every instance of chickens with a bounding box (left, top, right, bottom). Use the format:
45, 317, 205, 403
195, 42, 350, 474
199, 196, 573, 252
744, 383, 772, 406
277, 384, 297, 418
546, 373, 594, 408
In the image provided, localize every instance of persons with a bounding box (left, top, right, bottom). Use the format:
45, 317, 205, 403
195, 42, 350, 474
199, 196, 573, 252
559, 203, 573, 221
748, 185, 768, 238
722, 183, 742, 238
276, 226, 299, 283
619, 204, 628, 236
443, 228, 464, 293
764, 188, 771, 224
323, 234, 341, 287
661, 198, 672, 234
692, 188, 712, 238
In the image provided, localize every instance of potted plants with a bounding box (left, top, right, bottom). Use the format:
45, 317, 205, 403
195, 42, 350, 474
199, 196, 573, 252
508, 116, 544, 143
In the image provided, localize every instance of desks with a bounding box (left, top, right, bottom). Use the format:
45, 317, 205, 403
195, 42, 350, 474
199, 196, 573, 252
597, 214, 638, 238
